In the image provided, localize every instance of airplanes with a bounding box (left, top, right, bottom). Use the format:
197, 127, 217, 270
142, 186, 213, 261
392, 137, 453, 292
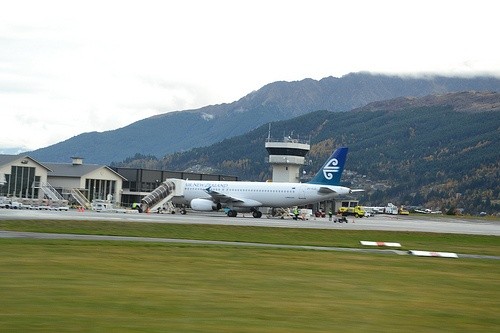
164, 146, 365, 218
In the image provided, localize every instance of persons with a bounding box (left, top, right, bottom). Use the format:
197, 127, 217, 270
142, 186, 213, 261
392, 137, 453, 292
293, 208, 300, 220
328, 207, 333, 218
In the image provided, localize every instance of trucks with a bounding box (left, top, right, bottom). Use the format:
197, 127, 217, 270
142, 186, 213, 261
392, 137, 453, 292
337, 206, 365, 218
